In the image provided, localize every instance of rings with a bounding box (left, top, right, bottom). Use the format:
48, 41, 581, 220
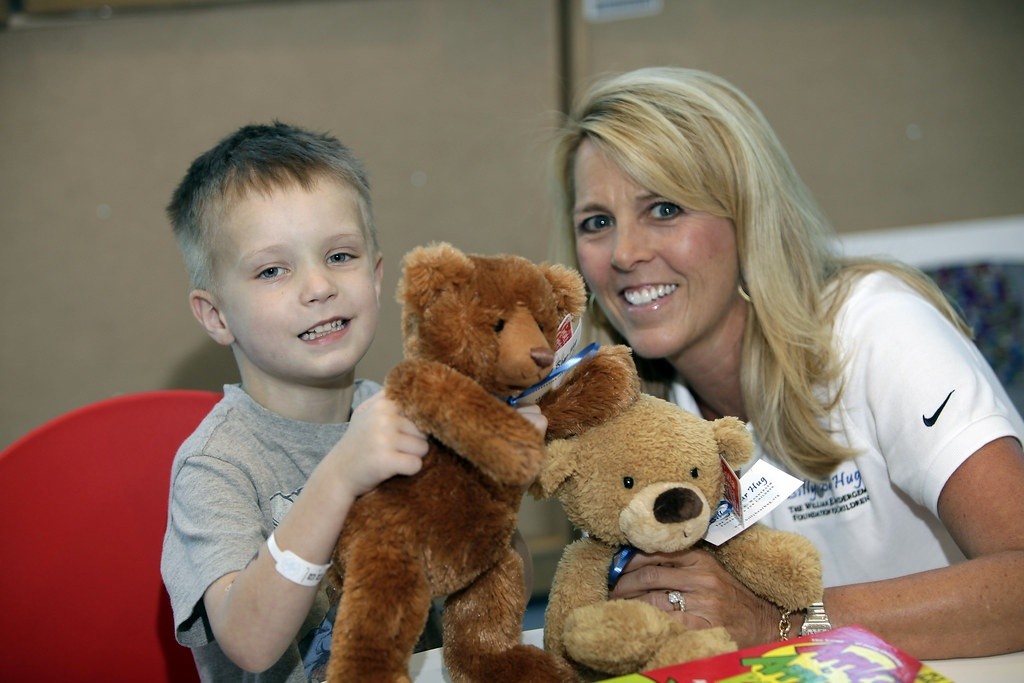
668, 590, 686, 612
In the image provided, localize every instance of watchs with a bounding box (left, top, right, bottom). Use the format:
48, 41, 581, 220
799, 600, 832, 638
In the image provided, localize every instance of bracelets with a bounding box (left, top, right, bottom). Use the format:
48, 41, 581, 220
779, 609, 792, 641
266, 531, 334, 587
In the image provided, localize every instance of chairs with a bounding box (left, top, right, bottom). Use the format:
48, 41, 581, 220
0, 388, 222, 679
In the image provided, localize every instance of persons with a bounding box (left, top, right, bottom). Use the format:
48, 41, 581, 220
559, 64, 1024, 661
158, 120, 552, 683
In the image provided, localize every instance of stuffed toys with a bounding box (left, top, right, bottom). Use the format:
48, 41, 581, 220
528, 343, 824, 674
324, 240, 586, 683
